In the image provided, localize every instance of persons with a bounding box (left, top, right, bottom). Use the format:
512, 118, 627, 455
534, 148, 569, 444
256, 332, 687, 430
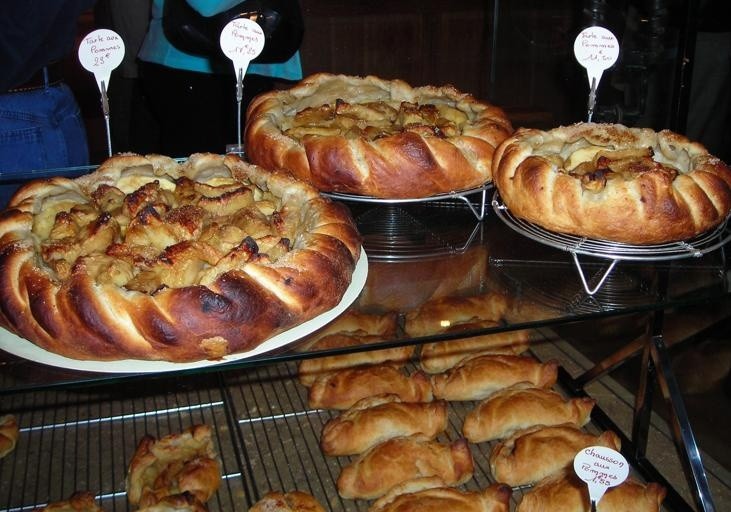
2, 2, 92, 210
135, 1, 304, 158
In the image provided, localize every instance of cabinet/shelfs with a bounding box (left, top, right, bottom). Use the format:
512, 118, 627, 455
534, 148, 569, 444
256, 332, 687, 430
0, 188, 731, 512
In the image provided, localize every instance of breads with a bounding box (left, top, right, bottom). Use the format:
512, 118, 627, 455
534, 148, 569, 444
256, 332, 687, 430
308, 363, 434, 410
320, 393, 448, 455
1, 413, 18, 455
244, 73, 516, 197
462, 380, 597, 443
490, 422, 621, 488
368, 474, 512, 512
140, 487, 211, 512
337, 433, 475, 500
45, 490, 105, 511
403, 290, 513, 338
492, 120, 727, 246
515, 475, 666, 512
420, 320, 531, 375
432, 354, 558, 402
246, 491, 329, 512
293, 309, 401, 352
124, 424, 223, 507
299, 329, 417, 387
